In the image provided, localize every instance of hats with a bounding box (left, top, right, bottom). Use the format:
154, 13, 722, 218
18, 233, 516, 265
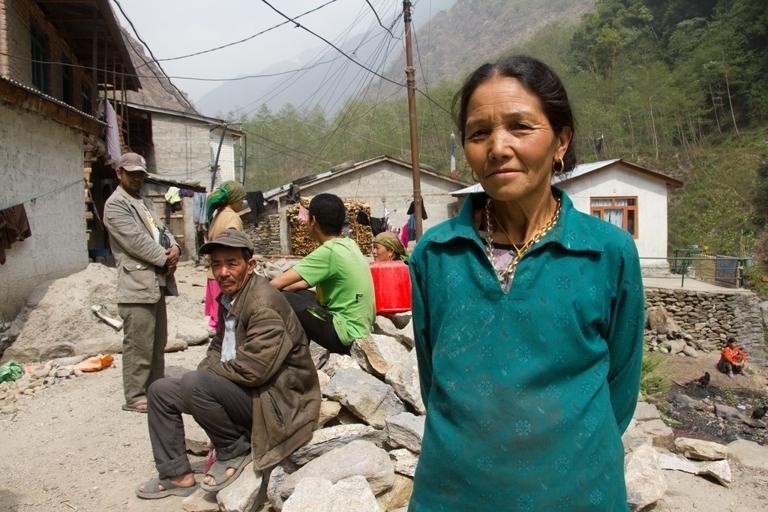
115, 151, 149, 175
197, 230, 254, 260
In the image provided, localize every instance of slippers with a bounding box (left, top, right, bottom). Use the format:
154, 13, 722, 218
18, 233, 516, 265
133, 476, 198, 500
201, 451, 253, 493
122, 398, 149, 413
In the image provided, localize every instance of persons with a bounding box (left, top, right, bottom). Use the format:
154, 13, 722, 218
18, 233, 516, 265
265, 194, 376, 356
135, 228, 322, 501
397, 53, 646, 512
196, 178, 247, 373
717, 336, 750, 381
101, 153, 181, 413
367, 231, 414, 316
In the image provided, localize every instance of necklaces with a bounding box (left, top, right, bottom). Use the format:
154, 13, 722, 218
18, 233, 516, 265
469, 193, 563, 287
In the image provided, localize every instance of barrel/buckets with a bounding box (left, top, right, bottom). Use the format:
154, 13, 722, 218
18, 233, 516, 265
371, 260, 412, 315
